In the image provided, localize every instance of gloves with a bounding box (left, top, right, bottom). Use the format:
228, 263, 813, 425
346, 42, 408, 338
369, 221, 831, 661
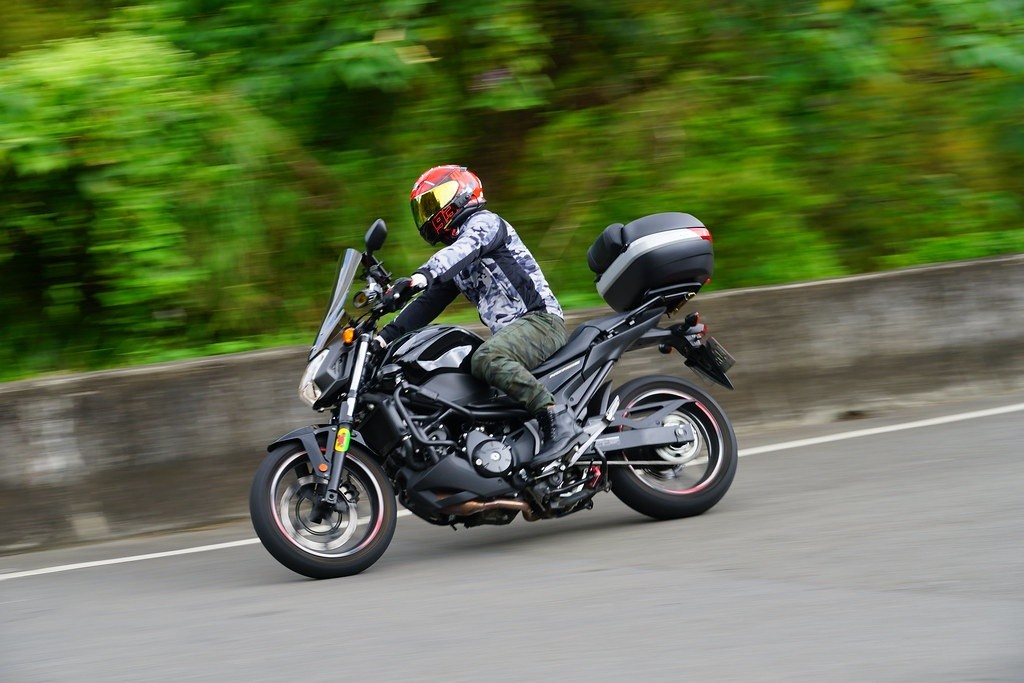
384, 273, 427, 313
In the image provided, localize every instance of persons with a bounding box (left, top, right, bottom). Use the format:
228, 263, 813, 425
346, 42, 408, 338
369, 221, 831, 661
368, 165, 589, 467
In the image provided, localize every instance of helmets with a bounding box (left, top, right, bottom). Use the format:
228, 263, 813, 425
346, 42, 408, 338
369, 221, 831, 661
410, 165, 487, 246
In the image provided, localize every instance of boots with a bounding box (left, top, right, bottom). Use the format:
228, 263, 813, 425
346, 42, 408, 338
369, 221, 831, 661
530, 404, 590, 467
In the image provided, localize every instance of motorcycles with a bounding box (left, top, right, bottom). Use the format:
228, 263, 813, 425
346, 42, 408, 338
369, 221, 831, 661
247, 210, 739, 581
